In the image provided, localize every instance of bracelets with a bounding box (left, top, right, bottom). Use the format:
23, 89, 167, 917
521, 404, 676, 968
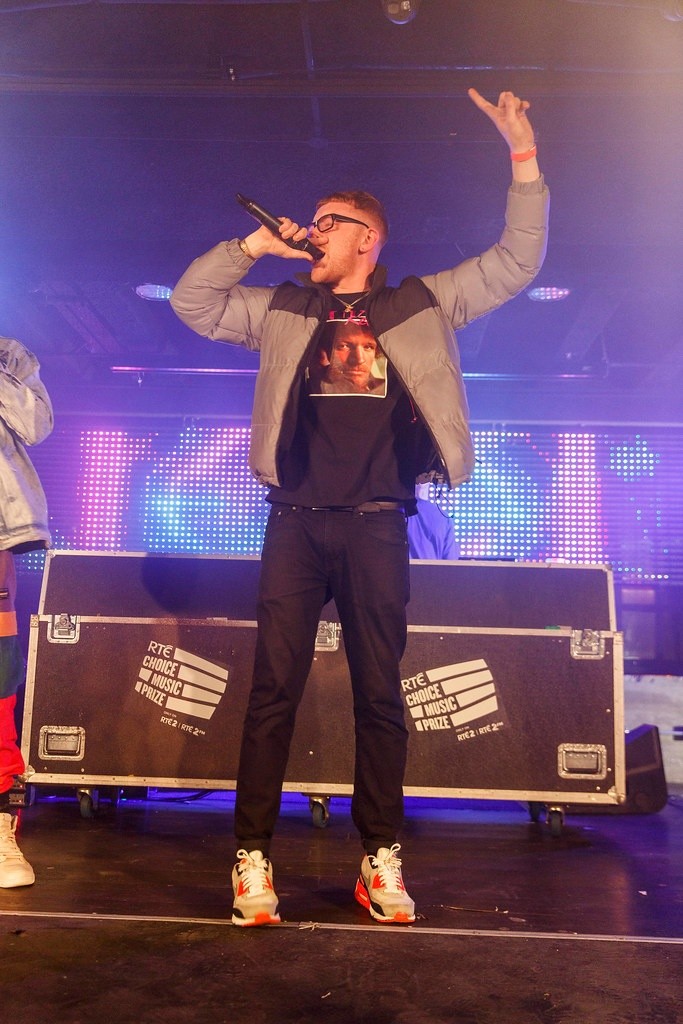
239, 240, 254, 260
510, 143, 538, 162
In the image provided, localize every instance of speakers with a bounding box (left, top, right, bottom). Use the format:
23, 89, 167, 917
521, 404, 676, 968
528, 723, 668, 819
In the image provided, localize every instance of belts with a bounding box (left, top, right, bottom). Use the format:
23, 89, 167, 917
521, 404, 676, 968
311, 500, 405, 514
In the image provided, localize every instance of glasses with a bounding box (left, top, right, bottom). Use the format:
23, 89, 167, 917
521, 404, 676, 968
304, 212, 369, 233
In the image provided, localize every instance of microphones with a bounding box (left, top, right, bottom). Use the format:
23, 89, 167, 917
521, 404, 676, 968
237, 193, 314, 253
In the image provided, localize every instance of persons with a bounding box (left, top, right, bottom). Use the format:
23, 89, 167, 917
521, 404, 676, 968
411, 496, 457, 562
172, 86, 549, 930
0, 333, 57, 892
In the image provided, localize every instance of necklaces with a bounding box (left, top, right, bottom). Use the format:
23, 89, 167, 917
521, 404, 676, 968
332, 289, 371, 313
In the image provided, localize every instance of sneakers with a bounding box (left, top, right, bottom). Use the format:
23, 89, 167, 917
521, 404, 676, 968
0, 813, 35, 888
232, 849, 282, 926
354, 843, 416, 923
